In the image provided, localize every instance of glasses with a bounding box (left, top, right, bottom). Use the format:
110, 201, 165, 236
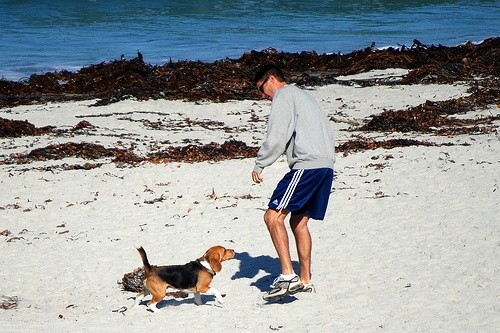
259, 76, 269, 92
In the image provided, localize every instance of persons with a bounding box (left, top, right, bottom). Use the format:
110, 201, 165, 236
250, 60, 336, 300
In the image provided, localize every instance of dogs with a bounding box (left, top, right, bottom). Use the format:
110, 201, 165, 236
130, 244, 235, 314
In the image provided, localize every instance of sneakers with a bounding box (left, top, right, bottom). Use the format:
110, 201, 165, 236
263, 272, 303, 301
302, 281, 316, 294
